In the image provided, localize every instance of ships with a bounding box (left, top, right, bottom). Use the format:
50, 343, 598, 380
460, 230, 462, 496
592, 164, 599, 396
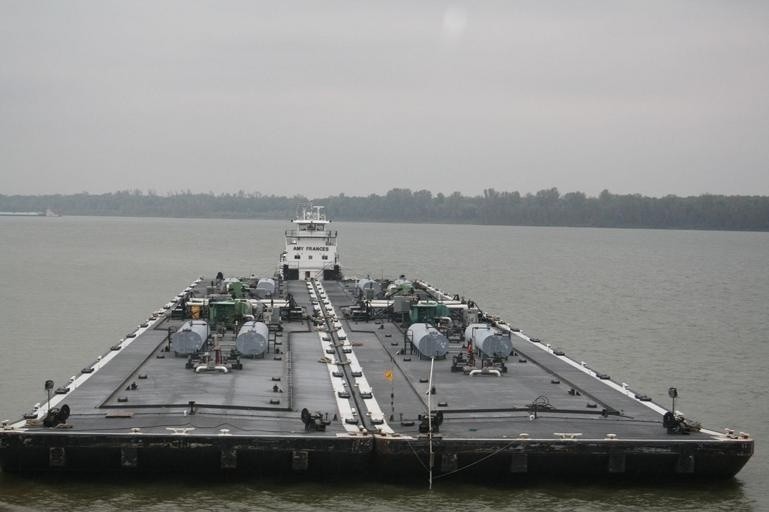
0, 202, 755, 492
